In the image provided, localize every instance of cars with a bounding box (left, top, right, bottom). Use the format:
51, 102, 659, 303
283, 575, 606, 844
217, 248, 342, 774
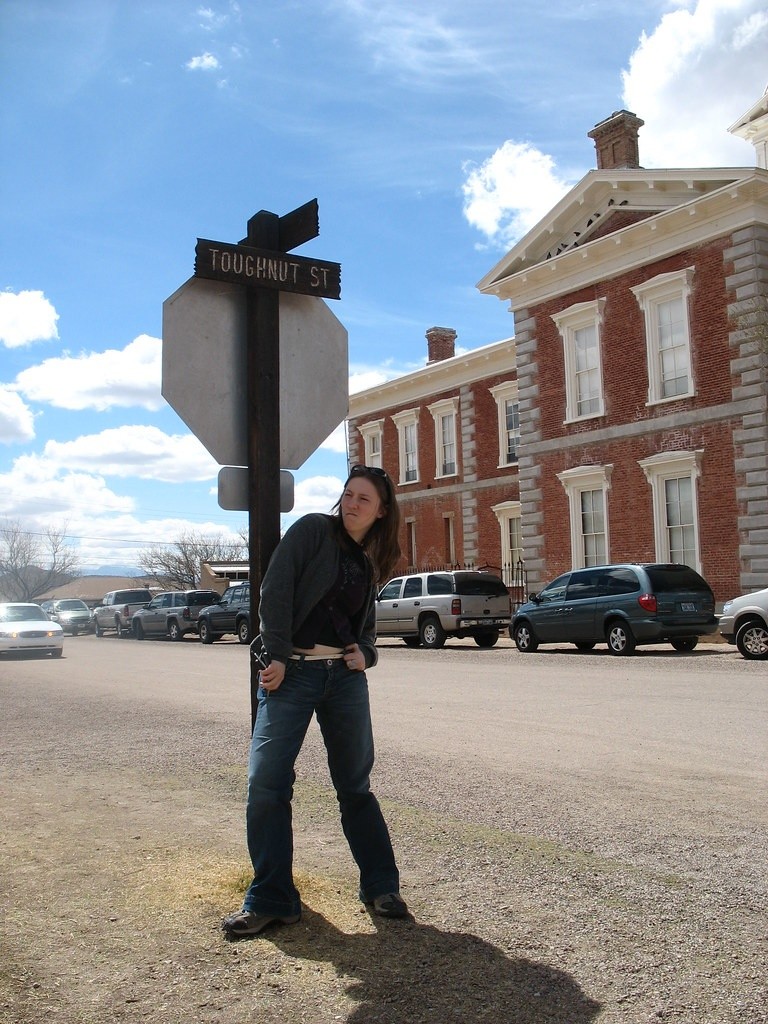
0, 602, 64, 657
719, 587, 768, 660
40, 599, 95, 636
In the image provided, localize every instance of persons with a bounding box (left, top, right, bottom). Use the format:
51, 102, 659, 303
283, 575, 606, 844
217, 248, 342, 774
224, 466, 408, 934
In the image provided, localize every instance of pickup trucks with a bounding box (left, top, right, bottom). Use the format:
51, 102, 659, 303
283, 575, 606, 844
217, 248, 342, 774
90, 588, 160, 639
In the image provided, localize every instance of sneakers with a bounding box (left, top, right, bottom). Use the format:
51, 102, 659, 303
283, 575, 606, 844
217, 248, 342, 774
222, 909, 300, 937
372, 891, 409, 920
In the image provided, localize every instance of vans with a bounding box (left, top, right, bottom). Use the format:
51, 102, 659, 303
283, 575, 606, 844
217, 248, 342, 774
509, 562, 718, 657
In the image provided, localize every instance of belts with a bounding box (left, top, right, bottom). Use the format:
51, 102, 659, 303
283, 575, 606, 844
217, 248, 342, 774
289, 652, 345, 661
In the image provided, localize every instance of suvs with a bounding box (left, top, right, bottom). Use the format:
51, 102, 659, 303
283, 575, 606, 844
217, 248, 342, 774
132, 588, 222, 642
197, 582, 252, 646
374, 570, 511, 650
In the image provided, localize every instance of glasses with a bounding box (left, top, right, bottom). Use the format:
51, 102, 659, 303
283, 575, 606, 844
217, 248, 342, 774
351, 464, 387, 481
